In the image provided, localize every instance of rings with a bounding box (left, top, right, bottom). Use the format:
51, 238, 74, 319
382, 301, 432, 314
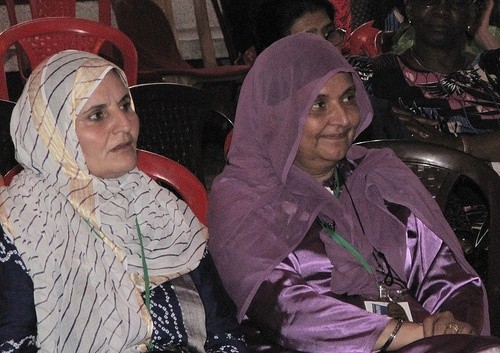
446, 321, 460, 334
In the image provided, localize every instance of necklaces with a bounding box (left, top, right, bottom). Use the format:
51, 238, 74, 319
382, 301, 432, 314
409, 46, 468, 76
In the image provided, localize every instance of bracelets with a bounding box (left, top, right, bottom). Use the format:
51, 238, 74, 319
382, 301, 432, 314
379, 318, 404, 352
460, 132, 472, 153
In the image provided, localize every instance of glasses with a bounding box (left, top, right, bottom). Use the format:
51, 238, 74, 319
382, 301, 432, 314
324, 25, 347, 46
418, 1, 477, 8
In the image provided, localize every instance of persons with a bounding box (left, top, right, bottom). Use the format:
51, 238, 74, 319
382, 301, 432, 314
224, 0, 500, 338
1, 49, 252, 353
207, 32, 500, 353
